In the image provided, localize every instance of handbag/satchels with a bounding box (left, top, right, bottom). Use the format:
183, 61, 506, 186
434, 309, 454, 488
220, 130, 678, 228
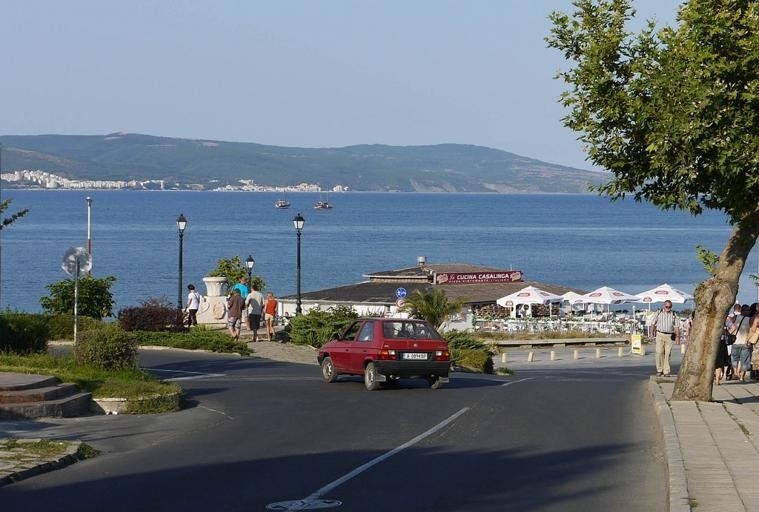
727, 334, 736, 345
747, 327, 759, 344
671, 333, 675, 340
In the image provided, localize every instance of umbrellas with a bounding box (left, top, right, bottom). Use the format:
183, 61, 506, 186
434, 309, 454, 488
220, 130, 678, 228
495, 281, 698, 331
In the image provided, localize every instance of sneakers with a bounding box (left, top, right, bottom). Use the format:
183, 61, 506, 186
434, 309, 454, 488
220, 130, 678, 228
656, 372, 662, 376
664, 374, 671, 377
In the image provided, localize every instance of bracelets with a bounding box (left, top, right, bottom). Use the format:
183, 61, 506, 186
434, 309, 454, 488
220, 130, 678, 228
675, 335, 679, 337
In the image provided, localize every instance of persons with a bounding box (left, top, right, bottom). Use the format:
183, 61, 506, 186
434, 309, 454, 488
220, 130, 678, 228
263, 292, 277, 341
685, 311, 694, 336
231, 276, 249, 300
651, 299, 680, 376
244, 285, 266, 341
285, 311, 289, 315
184, 284, 200, 328
227, 288, 246, 340
713, 302, 759, 385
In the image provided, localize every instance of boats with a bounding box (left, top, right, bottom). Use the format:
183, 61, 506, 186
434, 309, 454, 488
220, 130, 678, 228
274, 188, 291, 210
314, 186, 334, 211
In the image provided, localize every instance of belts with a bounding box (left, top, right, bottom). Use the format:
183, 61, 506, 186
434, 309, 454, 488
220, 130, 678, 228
656, 330, 674, 334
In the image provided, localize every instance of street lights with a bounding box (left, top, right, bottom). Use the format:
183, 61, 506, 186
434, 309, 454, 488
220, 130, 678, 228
243, 252, 255, 294
174, 211, 188, 332
291, 211, 305, 317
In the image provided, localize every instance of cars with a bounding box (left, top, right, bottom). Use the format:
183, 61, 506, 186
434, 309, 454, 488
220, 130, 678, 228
314, 314, 454, 392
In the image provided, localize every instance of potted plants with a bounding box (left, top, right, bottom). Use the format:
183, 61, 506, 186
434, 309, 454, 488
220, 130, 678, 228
202, 267, 231, 297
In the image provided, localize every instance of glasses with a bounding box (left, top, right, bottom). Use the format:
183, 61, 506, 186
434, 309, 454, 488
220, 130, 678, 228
665, 306, 671, 308
